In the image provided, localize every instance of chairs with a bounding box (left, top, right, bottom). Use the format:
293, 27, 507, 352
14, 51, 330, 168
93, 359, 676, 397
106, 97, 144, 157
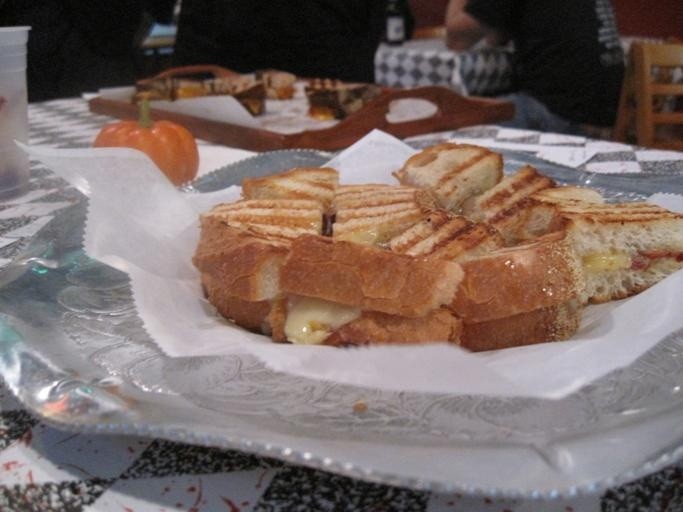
613, 41, 671, 144
632, 42, 683, 153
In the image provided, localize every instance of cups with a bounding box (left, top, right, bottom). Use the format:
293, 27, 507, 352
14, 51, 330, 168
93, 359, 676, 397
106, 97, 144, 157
0, 24, 32, 205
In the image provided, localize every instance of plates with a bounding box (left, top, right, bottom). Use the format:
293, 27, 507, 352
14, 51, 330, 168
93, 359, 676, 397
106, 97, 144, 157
0, 148, 683, 499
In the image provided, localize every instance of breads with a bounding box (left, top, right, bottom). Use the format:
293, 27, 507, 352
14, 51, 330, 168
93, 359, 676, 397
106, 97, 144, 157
190, 141, 683, 354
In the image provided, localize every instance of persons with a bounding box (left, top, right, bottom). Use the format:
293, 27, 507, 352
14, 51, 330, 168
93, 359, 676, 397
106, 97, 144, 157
443, 0, 625, 135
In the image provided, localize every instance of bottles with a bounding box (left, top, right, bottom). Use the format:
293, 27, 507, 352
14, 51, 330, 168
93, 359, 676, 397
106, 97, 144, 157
384, 0, 407, 45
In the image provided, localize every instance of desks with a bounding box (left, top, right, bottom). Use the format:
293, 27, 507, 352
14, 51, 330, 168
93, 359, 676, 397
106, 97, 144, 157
373, 39, 523, 98
142, 37, 176, 76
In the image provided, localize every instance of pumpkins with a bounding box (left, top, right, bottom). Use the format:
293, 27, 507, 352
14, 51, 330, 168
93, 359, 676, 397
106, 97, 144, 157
91, 99, 200, 187
